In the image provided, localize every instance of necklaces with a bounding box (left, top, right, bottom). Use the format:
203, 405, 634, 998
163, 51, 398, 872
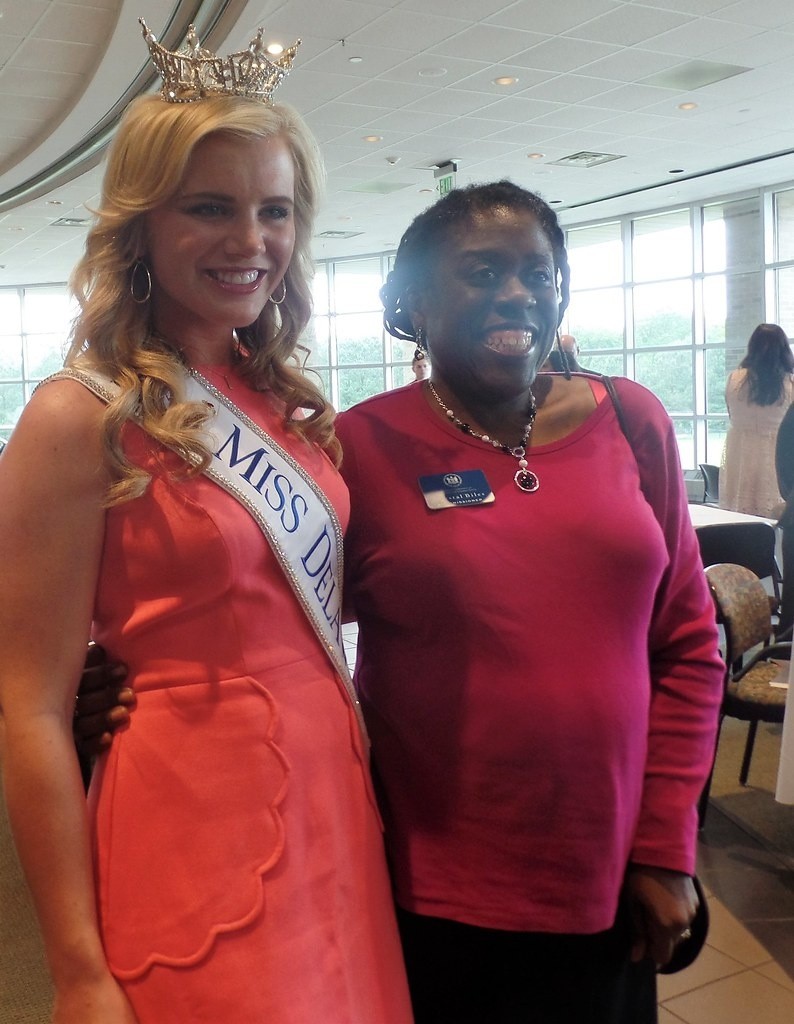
426, 374, 541, 492
155, 322, 246, 390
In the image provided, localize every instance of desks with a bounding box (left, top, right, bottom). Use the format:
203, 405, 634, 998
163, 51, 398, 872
688, 504, 779, 531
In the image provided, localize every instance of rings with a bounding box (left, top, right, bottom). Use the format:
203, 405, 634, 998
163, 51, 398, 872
680, 927, 691, 938
73, 695, 79, 716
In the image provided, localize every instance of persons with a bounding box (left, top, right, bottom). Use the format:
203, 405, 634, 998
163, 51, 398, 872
722, 322, 794, 650
538, 333, 600, 378
0, 84, 421, 1023
408, 352, 433, 383
69, 181, 727, 1024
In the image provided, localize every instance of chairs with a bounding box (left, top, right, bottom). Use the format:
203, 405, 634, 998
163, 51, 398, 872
699, 461, 794, 832
